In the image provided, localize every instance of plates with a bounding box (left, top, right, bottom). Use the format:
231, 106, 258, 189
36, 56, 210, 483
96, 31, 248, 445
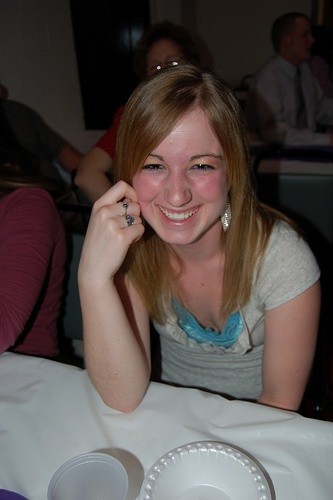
138, 440, 271, 500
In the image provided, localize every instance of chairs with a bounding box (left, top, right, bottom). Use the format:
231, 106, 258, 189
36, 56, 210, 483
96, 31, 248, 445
0, 159, 333, 418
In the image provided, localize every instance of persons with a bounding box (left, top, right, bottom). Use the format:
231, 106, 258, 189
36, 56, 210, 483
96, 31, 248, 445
0, 84, 88, 204
78, 64, 320, 416
245, 12, 333, 162
69, 22, 197, 202
0, 186, 68, 364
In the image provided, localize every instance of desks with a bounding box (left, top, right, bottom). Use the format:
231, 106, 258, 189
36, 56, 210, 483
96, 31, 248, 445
0, 351, 333, 500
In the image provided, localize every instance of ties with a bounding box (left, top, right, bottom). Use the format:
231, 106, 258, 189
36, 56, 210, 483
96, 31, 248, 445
292, 65, 308, 131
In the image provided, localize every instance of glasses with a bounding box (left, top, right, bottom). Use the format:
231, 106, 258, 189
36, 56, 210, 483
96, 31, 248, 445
144, 58, 184, 74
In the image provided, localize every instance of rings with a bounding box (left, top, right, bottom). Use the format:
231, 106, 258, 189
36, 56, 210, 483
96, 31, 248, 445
125, 215, 134, 226
123, 202, 129, 216
